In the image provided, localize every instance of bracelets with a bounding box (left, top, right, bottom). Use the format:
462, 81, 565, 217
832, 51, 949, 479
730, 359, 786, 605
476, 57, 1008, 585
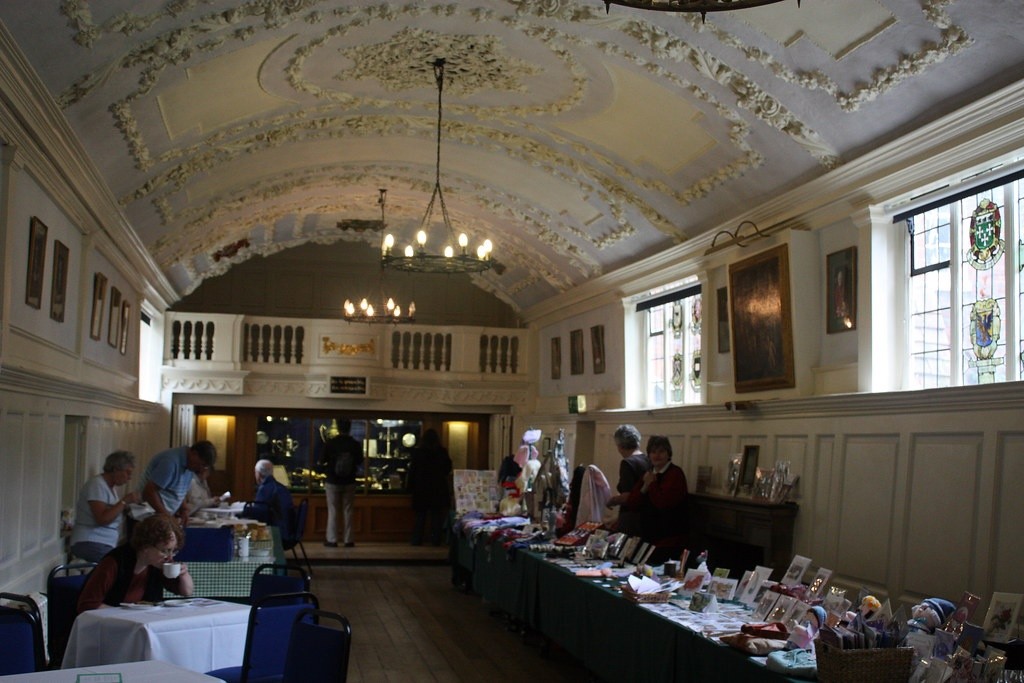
120, 500, 127, 507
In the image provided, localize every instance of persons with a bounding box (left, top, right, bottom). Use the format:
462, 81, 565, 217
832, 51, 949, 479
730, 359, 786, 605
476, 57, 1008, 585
235, 460, 295, 550
906, 598, 955, 634
606, 424, 692, 565
319, 414, 364, 546
127, 441, 220, 542
71, 450, 142, 566
75, 513, 193, 614
782, 606, 827, 654
408, 429, 453, 547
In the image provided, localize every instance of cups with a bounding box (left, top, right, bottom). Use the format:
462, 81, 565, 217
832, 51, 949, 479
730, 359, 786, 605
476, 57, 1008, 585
237, 537, 249, 556
162, 562, 181, 579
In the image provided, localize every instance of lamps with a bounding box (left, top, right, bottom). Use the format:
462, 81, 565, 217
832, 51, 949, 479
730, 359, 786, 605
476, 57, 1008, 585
342, 189, 417, 325
381, 56, 500, 276
703, 221, 770, 257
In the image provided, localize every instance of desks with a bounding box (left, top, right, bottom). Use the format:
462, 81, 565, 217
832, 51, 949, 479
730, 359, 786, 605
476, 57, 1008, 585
60, 598, 252, 673
195, 507, 237, 518
163, 525, 276, 603
0, 659, 227, 683
687, 489, 801, 582
444, 514, 820, 683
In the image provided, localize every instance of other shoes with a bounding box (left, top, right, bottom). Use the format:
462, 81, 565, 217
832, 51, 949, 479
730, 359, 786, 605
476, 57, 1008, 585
345, 542, 355, 547
325, 541, 338, 547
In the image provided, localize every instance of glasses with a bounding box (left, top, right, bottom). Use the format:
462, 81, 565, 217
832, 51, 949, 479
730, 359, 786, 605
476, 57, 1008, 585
153, 545, 180, 557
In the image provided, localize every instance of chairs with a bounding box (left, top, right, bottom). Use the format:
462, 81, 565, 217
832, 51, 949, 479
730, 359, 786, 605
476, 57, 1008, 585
0, 590, 47, 676
203, 590, 321, 683
47, 562, 99, 658
282, 607, 352, 683
282, 496, 314, 577
250, 564, 311, 607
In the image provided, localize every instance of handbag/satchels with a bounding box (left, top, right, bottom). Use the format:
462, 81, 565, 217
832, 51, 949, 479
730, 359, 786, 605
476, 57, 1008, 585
173, 524, 234, 562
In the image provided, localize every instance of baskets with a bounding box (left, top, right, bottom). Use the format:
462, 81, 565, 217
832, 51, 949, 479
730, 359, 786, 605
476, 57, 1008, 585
814, 639, 915, 683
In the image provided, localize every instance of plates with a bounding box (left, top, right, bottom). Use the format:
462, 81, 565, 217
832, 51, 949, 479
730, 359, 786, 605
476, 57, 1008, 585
251, 549, 269, 557
119, 602, 163, 610
164, 599, 193, 607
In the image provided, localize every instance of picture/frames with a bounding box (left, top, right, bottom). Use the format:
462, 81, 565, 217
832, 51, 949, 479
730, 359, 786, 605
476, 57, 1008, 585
119, 298, 130, 356
784, 600, 811, 629
49, 239, 70, 323
90, 272, 109, 342
107, 285, 122, 349
714, 578, 738, 600
570, 329, 584, 376
781, 554, 812, 586
677, 568, 708, 597
551, 336, 561, 380
765, 594, 798, 624
804, 567, 833, 600
735, 570, 752, 598
826, 245, 857, 335
25, 216, 49, 310
590, 324, 605, 375
728, 242, 796, 394
740, 444, 760, 486
982, 592, 1024, 643
716, 286, 731, 355
753, 590, 779, 622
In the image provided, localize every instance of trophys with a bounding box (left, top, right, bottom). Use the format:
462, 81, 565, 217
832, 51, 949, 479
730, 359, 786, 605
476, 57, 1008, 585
276, 434, 299, 457
319, 417, 339, 443
